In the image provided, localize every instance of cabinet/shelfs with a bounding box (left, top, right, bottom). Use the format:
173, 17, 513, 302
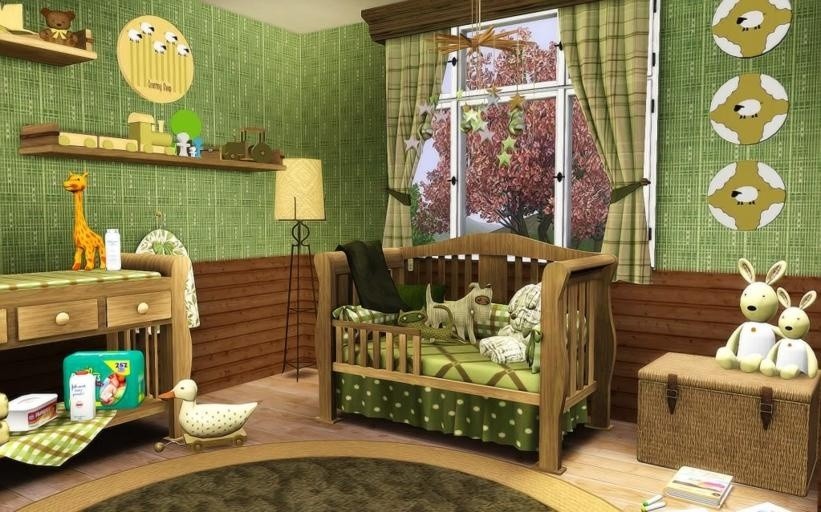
0, 251, 195, 443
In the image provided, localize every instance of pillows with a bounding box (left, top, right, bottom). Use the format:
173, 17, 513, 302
390, 281, 450, 312
331, 301, 399, 345
522, 309, 589, 376
440, 300, 511, 339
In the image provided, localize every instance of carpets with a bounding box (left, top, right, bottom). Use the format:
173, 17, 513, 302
12, 440, 623, 512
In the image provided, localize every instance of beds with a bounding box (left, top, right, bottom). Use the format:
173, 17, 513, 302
312, 231, 620, 476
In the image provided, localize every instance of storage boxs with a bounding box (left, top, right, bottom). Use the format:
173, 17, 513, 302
635, 350, 817, 496
4, 392, 59, 432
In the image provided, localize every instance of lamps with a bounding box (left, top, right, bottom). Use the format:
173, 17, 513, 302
273, 156, 325, 383
